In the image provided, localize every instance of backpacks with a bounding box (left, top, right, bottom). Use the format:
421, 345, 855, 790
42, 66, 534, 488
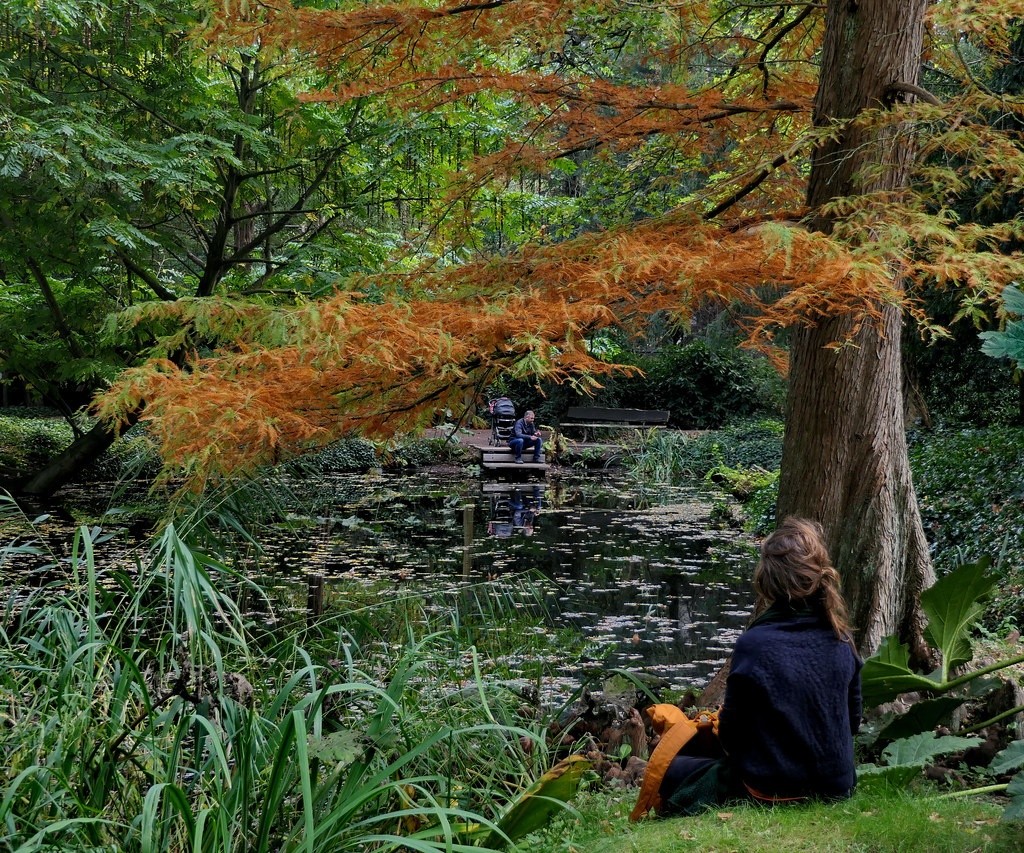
628, 699, 719, 823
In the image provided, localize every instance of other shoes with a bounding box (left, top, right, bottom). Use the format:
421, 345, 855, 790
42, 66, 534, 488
514, 458, 523, 463
533, 459, 545, 463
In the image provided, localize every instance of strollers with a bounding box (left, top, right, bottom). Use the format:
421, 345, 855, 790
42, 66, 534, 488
487, 397, 516, 447
487, 500, 515, 539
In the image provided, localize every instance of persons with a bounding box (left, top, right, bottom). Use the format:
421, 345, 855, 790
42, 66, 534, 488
511, 486, 544, 540
653, 516, 862, 817
507, 410, 546, 464
